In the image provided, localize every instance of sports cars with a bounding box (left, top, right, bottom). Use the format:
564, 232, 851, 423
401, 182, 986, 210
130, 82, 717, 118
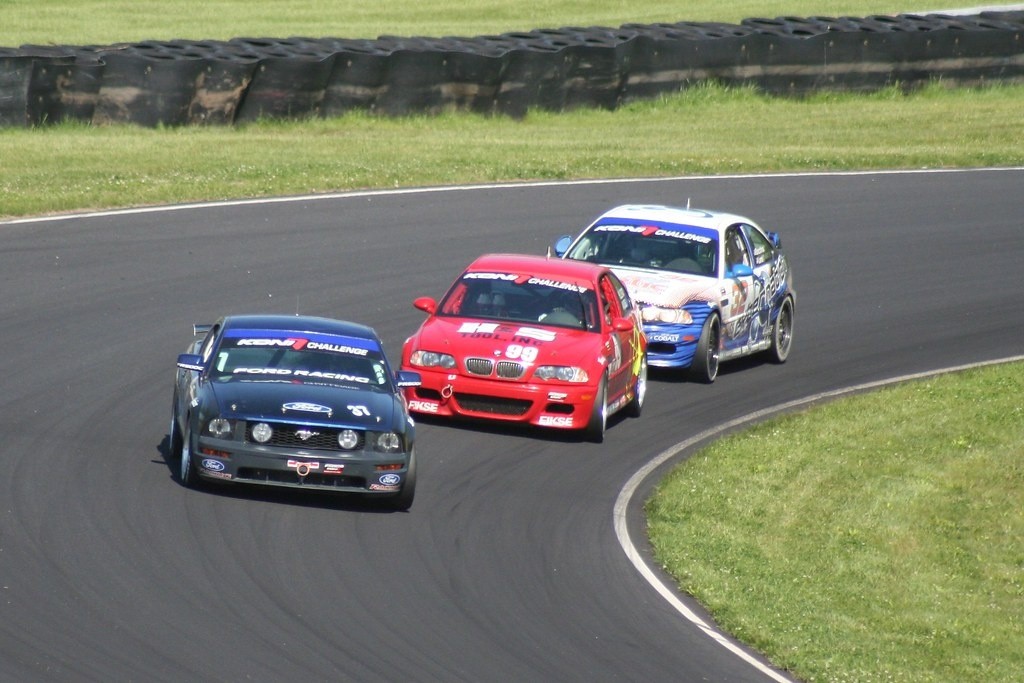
553, 202, 800, 385
168, 311, 423, 513
399, 252, 650, 441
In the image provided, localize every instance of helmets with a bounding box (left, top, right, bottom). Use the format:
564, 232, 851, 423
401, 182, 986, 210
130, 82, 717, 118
693, 245, 714, 266
